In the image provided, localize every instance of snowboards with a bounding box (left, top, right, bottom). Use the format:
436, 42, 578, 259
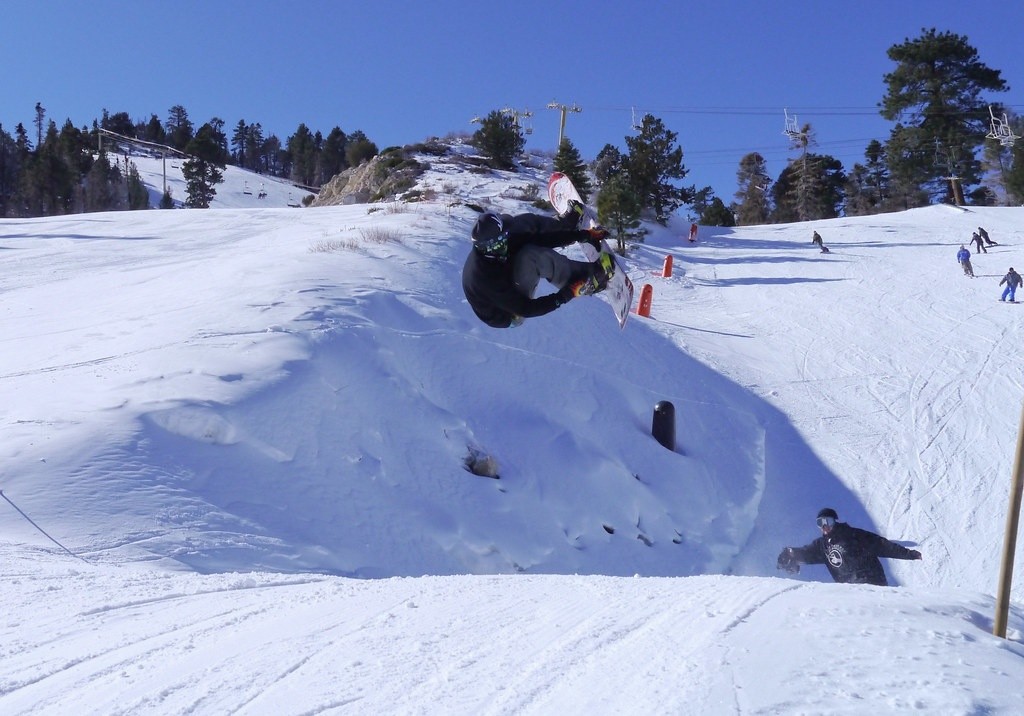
964, 273, 978, 279
547, 171, 634, 331
972, 251, 993, 255
820, 251, 831, 254
984, 244, 1000, 248
996, 299, 1024, 304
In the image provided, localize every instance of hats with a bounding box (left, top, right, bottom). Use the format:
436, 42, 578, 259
471, 213, 513, 244
817, 508, 838, 519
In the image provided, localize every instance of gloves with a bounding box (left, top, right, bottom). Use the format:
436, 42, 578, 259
586, 227, 611, 252
559, 272, 591, 304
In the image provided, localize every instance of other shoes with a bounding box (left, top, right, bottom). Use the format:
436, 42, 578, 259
990, 242, 997, 246
976, 251, 980, 254
970, 273, 974, 276
983, 251, 987, 253
999, 298, 1006, 302
966, 272, 969, 275
1009, 299, 1015, 301
824, 249, 829, 253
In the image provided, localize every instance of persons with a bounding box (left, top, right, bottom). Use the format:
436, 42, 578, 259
999, 267, 1022, 301
461, 199, 611, 329
812, 231, 829, 253
784, 507, 922, 587
957, 244, 974, 277
970, 232, 987, 254
978, 227, 997, 246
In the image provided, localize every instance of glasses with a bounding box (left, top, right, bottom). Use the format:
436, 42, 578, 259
816, 516, 835, 528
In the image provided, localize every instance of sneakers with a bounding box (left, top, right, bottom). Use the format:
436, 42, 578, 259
558, 199, 584, 244
591, 252, 615, 293
